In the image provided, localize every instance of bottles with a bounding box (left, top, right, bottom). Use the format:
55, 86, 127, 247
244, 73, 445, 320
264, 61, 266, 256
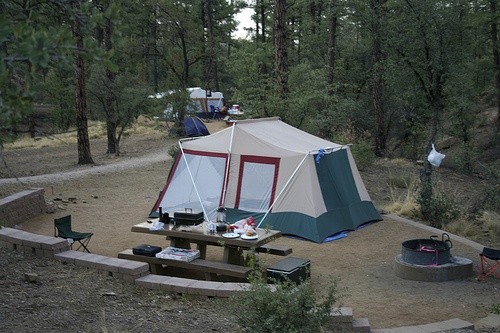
163, 211, 169, 224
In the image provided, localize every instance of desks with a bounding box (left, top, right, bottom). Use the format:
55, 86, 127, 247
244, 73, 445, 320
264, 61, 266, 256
130, 217, 282, 283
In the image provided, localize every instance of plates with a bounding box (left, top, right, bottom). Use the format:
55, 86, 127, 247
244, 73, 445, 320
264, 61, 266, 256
241, 234, 258, 240
222, 232, 240, 238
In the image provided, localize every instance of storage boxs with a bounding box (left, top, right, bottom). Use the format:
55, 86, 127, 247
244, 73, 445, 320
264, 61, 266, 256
265, 256, 312, 291
174, 207, 204, 227
132, 244, 162, 257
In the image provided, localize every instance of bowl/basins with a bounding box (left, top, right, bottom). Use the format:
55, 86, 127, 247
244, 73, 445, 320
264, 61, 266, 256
216, 225, 226, 233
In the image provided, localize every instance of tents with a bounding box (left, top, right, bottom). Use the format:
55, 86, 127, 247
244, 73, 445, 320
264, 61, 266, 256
171, 112, 210, 138
148, 115, 385, 246
148, 85, 227, 118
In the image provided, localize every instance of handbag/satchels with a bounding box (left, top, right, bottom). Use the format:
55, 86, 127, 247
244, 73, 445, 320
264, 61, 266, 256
427, 143, 446, 167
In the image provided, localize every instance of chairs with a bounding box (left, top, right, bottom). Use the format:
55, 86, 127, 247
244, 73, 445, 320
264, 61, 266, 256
53, 214, 94, 255
477, 247, 500, 281
117, 248, 253, 281
209, 105, 222, 120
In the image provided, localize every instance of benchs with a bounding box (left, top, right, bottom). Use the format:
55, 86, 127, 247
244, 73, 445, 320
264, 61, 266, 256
166, 235, 293, 261
